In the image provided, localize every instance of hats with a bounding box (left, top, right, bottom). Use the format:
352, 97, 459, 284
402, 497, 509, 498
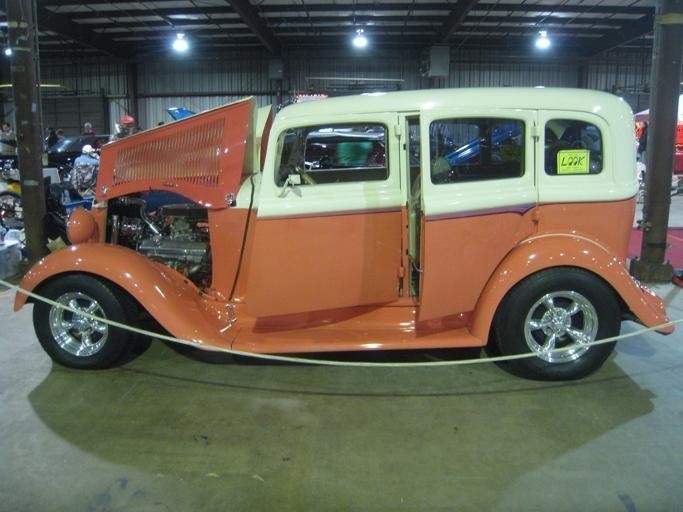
81, 116, 135, 154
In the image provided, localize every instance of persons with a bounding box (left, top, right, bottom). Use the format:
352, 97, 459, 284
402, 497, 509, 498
72, 143, 100, 168
80, 121, 96, 136
0, 121, 11, 135
45, 124, 57, 147
110, 114, 144, 141
53, 128, 64, 140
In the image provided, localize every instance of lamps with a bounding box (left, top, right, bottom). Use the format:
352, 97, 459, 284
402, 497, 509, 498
352, 28, 369, 49
535, 29, 550, 49
171, 32, 189, 54
